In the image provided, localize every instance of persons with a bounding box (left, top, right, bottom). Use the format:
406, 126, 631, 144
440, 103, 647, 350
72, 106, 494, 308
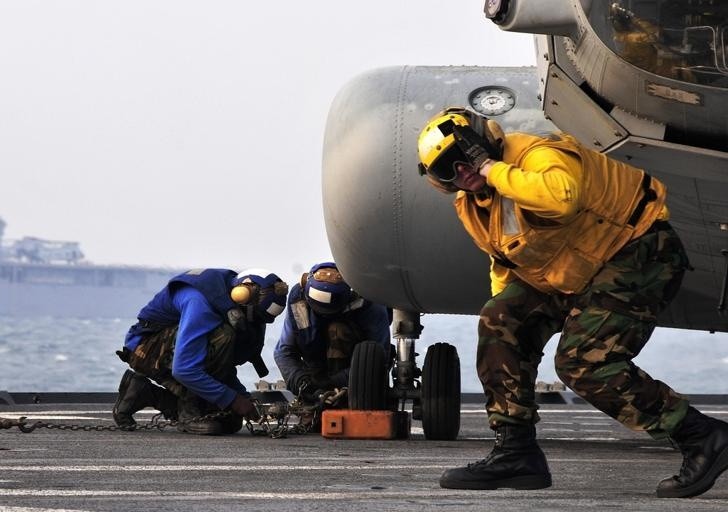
272, 261, 395, 435
415, 105, 728, 500
112, 267, 289, 438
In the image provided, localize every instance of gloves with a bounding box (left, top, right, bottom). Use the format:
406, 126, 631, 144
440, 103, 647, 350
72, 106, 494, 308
229, 394, 260, 423
451, 123, 491, 173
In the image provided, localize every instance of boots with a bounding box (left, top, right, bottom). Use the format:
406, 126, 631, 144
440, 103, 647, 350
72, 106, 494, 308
111, 369, 176, 432
654, 404, 728, 499
438, 420, 552, 492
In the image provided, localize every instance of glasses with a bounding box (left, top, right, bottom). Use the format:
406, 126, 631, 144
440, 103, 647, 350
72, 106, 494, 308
430, 141, 470, 183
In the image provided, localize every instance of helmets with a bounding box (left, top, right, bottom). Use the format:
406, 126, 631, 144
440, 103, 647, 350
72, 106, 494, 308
416, 105, 507, 195
230, 268, 287, 325
300, 262, 351, 315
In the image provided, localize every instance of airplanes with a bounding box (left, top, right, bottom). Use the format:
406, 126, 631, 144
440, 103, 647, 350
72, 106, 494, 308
316, 0, 728, 451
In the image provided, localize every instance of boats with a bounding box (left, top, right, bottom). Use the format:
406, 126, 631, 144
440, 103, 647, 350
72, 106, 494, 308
0, 220, 191, 322
